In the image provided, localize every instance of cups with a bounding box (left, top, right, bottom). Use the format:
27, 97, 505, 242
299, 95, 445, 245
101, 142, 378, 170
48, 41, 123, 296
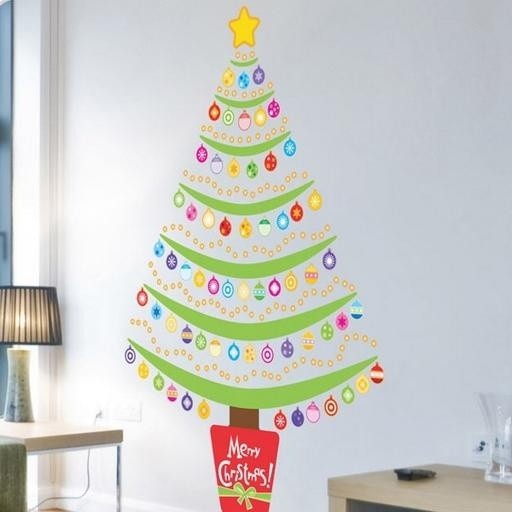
471, 387, 512, 486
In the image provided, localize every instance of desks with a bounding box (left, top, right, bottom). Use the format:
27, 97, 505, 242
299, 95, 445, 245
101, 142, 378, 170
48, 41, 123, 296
0, 423, 124, 511
327, 464, 511, 512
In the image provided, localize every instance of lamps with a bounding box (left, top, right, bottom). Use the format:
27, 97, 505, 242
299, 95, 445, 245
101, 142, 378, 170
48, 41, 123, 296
0, 286, 62, 424
